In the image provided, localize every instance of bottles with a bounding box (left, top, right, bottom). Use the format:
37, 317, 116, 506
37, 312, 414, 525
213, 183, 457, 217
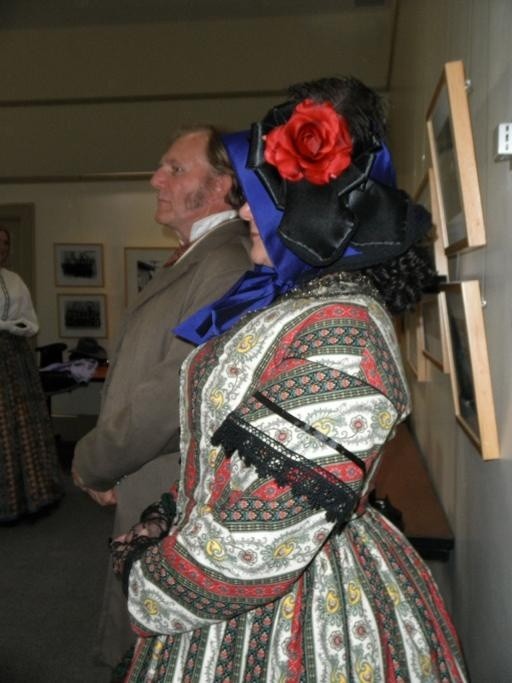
68, 336, 108, 360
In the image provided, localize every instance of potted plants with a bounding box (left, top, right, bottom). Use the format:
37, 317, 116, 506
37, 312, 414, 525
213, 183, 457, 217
50, 240, 178, 337
405, 57, 499, 462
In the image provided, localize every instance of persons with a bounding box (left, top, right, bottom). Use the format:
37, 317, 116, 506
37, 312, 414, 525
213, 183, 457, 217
70, 122, 248, 667
111, 76, 470, 683
0, 225, 66, 523
